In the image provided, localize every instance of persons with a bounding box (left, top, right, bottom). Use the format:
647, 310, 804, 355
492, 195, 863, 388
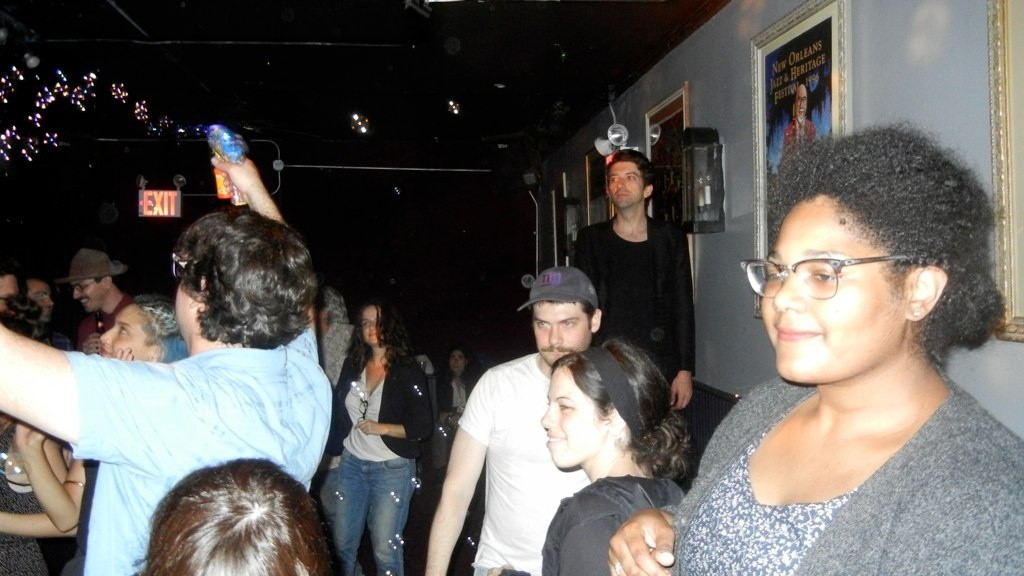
1, 157, 475, 576
488, 340, 698, 576
425, 268, 602, 576
606, 128, 1024, 575
575, 150, 696, 410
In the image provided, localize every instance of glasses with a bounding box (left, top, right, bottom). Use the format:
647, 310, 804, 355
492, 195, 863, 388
357, 399, 368, 423
71, 280, 99, 292
173, 252, 204, 290
740, 255, 908, 300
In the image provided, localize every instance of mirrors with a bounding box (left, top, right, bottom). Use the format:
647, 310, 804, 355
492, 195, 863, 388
645, 82, 695, 304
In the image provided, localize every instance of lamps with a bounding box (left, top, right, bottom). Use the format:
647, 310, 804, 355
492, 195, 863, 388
594, 123, 640, 157
681, 124, 724, 235
649, 125, 661, 146
135, 172, 185, 218
522, 168, 543, 190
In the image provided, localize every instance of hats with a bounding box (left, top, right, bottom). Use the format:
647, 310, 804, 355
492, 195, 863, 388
55, 248, 127, 283
517, 267, 598, 311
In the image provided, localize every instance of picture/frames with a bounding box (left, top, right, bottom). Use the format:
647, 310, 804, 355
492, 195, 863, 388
750, 0, 839, 319
987, 0, 1024, 343
585, 146, 614, 225
